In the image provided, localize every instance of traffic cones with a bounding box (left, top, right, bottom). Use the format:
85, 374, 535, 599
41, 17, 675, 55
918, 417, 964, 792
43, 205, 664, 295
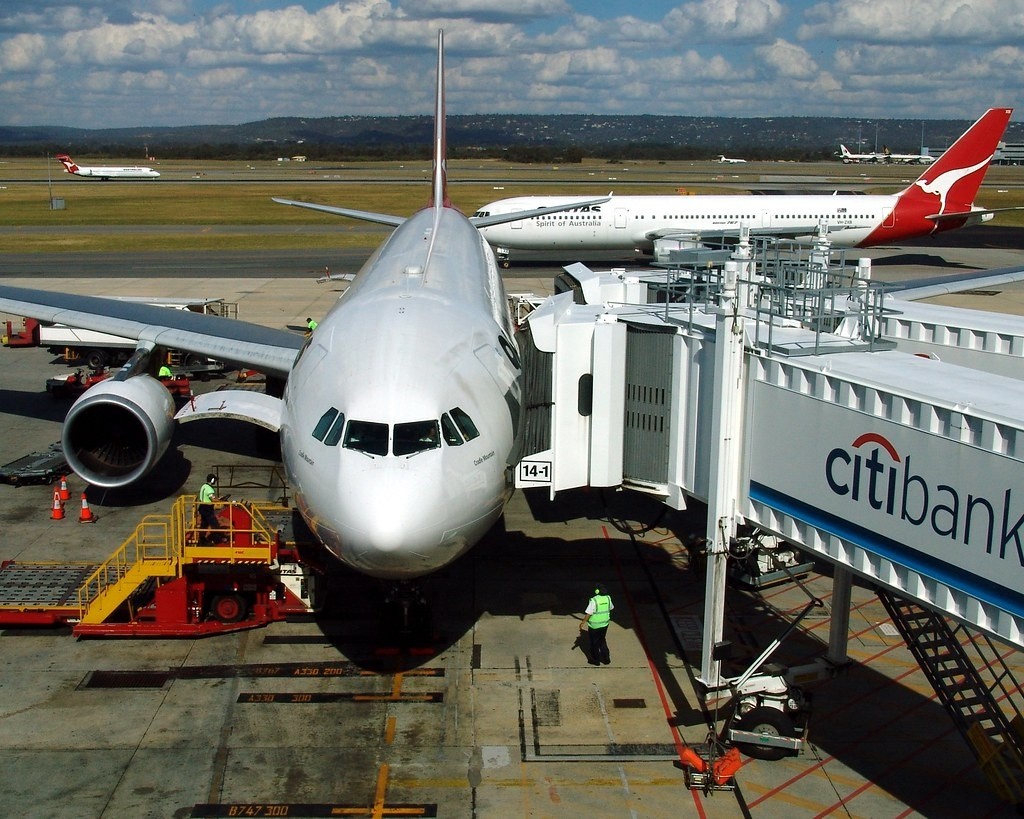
51, 486, 62, 510
79, 492, 96, 523
51, 494, 63, 519
59, 475, 70, 500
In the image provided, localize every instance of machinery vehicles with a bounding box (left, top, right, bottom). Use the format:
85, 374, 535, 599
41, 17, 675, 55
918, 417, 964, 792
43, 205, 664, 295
39, 295, 239, 372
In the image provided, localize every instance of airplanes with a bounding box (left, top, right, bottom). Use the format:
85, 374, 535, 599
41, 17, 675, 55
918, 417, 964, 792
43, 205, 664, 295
717, 155, 748, 164
0, 25, 1024, 590
56, 153, 162, 183
834, 143, 937, 165
470, 107, 1023, 261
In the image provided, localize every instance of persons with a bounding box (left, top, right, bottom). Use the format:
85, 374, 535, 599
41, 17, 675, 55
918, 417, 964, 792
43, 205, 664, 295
578, 583, 614, 666
158, 360, 174, 377
307, 318, 318, 332
198, 474, 229, 548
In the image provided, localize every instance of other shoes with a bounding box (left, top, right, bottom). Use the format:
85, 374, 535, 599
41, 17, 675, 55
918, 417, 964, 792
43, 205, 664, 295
600, 655, 611, 665
588, 658, 601, 666
